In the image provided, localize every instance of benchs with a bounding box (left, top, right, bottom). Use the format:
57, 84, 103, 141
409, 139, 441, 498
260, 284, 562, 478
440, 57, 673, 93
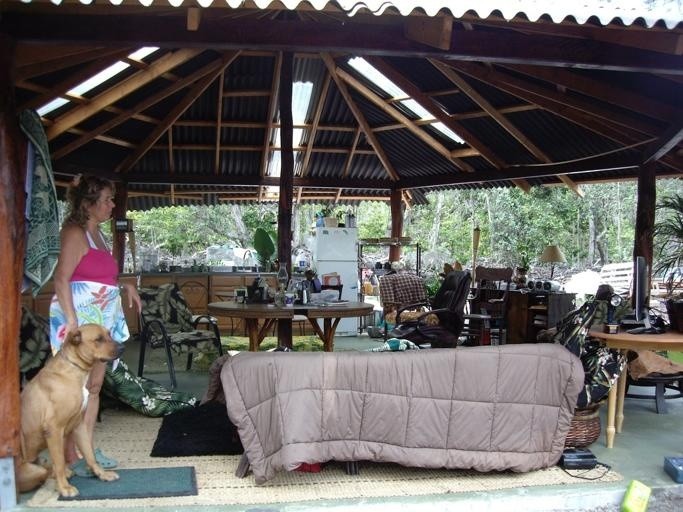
223, 343, 585, 478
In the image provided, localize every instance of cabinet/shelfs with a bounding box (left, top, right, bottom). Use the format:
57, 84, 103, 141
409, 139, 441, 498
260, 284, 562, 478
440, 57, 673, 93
466, 285, 576, 347
354, 241, 420, 335
118, 277, 278, 336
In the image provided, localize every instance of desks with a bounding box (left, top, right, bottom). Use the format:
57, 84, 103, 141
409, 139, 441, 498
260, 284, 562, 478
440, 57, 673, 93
207, 300, 374, 352
588, 329, 682, 448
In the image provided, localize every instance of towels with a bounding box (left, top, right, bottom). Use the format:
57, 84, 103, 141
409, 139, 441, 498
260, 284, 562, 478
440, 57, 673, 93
16, 105, 60, 301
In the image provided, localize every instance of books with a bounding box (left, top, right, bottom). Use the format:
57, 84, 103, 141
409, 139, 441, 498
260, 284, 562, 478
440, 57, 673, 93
309, 302, 349, 306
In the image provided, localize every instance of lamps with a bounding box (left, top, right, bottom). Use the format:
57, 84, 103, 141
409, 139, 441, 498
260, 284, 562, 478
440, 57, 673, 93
537, 244, 567, 292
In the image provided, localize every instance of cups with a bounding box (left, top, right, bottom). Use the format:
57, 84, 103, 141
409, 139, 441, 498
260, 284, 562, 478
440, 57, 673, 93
284, 293, 293, 308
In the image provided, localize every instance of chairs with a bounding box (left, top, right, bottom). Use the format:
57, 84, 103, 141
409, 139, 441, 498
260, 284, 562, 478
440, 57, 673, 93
379, 264, 513, 350
131, 282, 222, 390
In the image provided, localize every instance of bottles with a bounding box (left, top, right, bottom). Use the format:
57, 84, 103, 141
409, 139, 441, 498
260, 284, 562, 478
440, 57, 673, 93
526, 276, 560, 292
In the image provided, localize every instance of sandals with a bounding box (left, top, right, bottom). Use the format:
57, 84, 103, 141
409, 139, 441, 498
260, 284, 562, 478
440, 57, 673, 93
69, 458, 96, 478
94, 448, 118, 468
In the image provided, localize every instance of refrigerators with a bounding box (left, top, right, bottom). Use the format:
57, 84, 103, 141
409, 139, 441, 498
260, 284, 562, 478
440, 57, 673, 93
310, 227, 359, 337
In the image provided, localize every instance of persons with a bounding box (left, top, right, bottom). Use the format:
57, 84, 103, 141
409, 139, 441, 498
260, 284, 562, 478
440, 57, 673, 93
440, 262, 452, 278
537, 284, 613, 343
452, 260, 462, 270
48, 171, 141, 479
386, 261, 400, 276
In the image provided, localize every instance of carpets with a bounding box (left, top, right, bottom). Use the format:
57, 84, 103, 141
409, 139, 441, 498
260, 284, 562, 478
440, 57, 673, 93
26, 407, 625, 507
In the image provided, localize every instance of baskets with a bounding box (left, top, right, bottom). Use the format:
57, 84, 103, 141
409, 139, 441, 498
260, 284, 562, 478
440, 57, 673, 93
566, 402, 601, 446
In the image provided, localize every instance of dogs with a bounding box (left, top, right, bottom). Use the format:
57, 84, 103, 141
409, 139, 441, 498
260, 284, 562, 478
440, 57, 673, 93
12, 322, 127, 498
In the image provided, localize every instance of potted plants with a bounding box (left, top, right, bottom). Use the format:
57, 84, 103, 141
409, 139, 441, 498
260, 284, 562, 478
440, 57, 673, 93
645, 192, 683, 334
320, 208, 346, 227
